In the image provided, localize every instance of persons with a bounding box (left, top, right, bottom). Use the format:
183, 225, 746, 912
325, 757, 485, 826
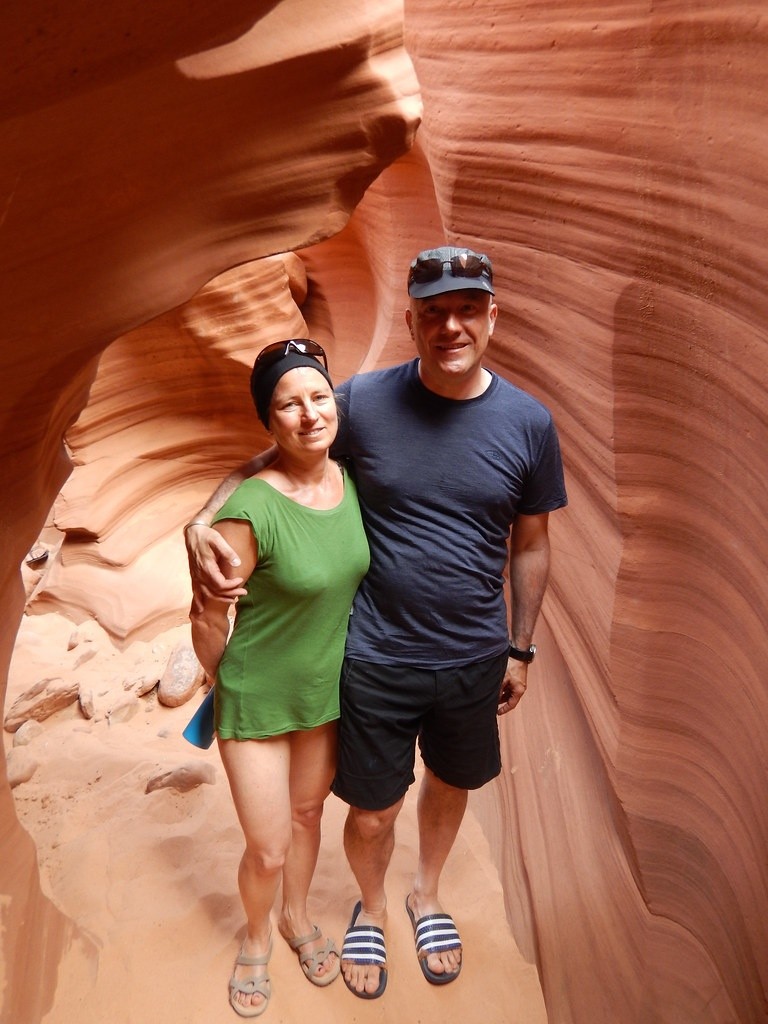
182, 247, 569, 999
189, 339, 370, 1017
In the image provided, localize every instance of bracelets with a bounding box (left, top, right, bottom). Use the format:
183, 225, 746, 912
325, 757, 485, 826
183, 521, 211, 531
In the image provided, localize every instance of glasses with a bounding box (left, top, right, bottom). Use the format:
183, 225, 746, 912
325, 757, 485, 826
250, 338, 328, 390
408, 255, 493, 296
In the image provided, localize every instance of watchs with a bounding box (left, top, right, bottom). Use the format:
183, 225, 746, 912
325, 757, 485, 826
509, 640, 537, 664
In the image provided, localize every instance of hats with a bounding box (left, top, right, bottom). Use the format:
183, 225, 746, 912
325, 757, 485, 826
407, 246, 496, 299
250, 347, 335, 431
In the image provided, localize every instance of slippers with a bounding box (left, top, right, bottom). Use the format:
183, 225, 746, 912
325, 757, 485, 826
405, 892, 463, 986
340, 900, 388, 1000
277, 921, 341, 987
229, 935, 272, 1018
